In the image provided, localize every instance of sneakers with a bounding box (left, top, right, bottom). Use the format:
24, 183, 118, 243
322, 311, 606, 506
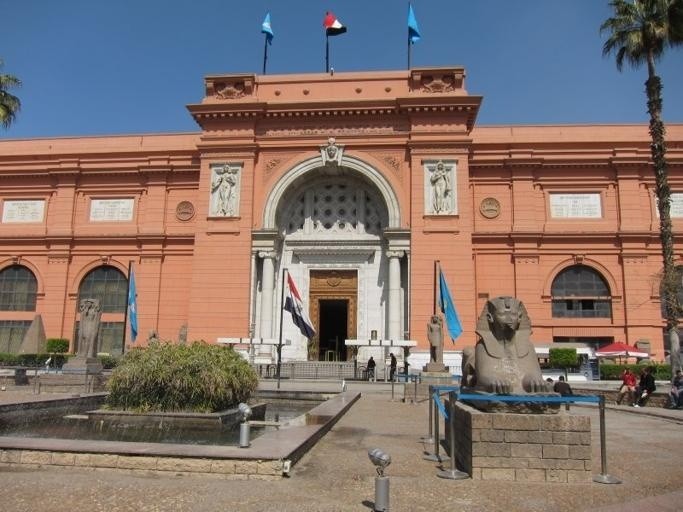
633, 403, 677, 408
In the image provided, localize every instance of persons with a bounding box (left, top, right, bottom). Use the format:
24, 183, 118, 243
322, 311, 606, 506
326, 146, 339, 166
430, 161, 451, 214
387, 353, 397, 381
616, 368, 637, 407
546, 376, 573, 410
211, 165, 236, 215
427, 314, 444, 364
78, 298, 102, 359
669, 370, 683, 409
635, 367, 656, 407
368, 357, 376, 381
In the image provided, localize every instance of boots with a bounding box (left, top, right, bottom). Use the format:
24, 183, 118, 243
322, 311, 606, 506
615, 392, 625, 405
629, 391, 636, 406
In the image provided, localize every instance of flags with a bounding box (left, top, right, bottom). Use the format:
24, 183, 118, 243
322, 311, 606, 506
438, 268, 464, 345
322, 12, 346, 36
284, 271, 318, 343
128, 266, 138, 342
262, 12, 274, 46
407, 4, 422, 46
